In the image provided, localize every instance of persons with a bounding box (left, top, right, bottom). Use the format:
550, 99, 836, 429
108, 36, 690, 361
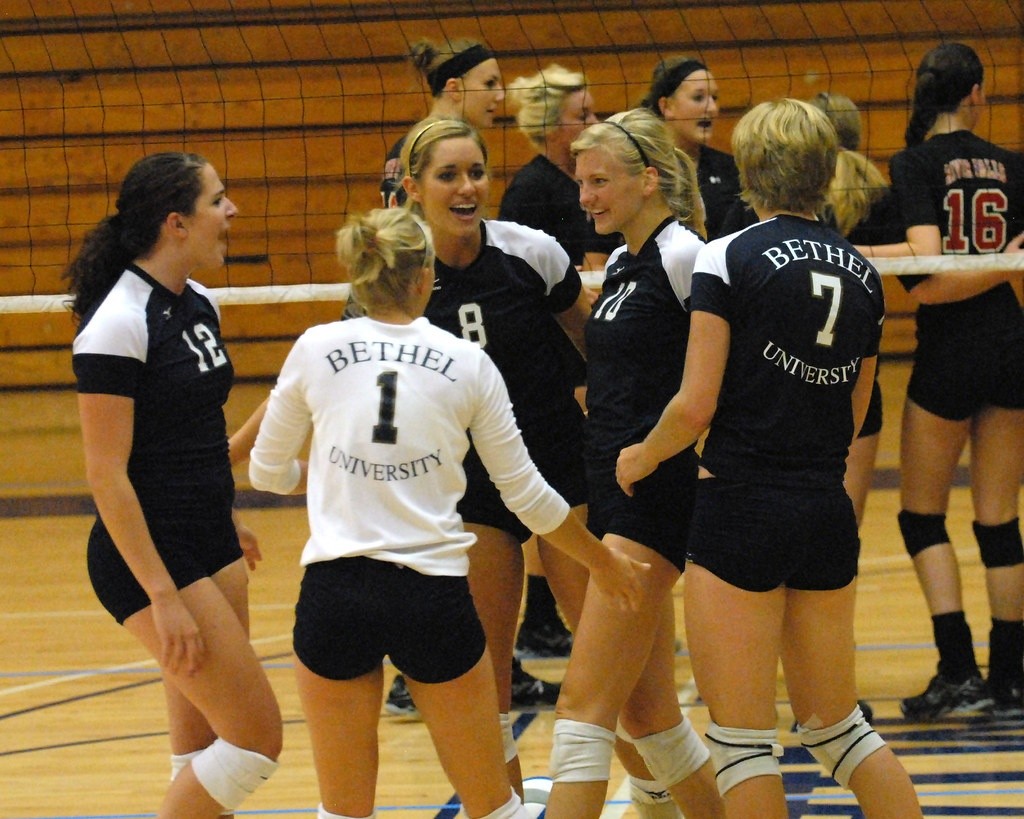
502, 61, 629, 657
635, 52, 762, 247
802, 86, 895, 524
610, 94, 927, 818
380, 33, 559, 710
247, 203, 652, 817
542, 107, 728, 819
851, 43, 1024, 722
225, 113, 680, 819
63, 151, 286, 818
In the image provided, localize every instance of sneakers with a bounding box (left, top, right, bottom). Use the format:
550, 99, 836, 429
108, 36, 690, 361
384, 675, 420, 713
982, 670, 1024, 718
514, 618, 574, 659
509, 655, 562, 711
900, 666, 995, 719
